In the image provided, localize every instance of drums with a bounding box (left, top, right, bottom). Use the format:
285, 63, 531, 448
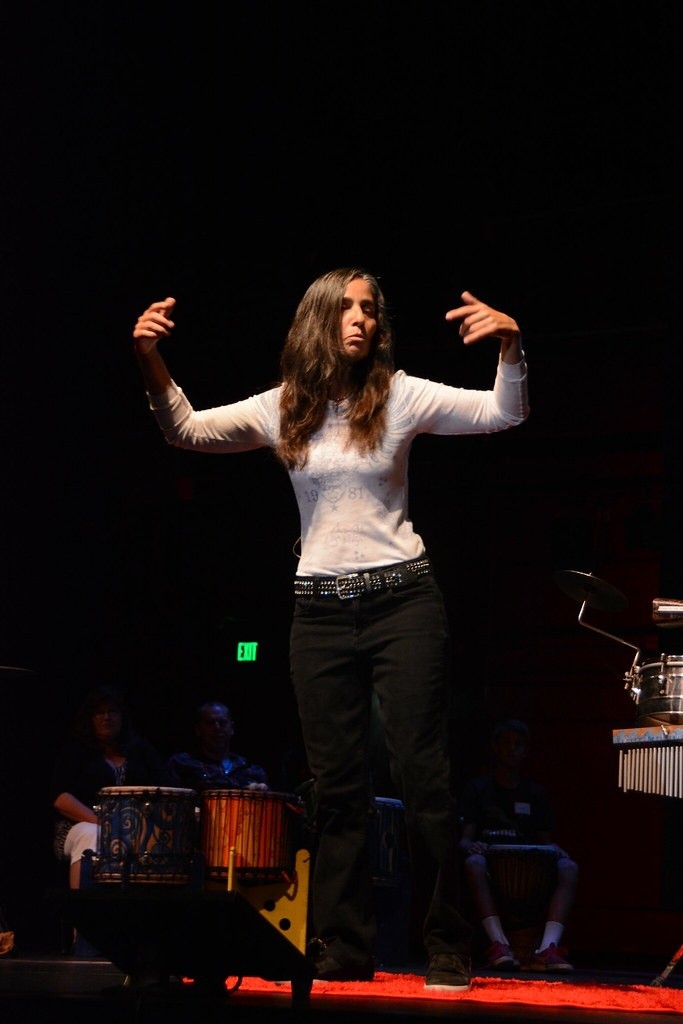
622, 653, 683, 728
199, 788, 296, 900
93, 785, 206, 890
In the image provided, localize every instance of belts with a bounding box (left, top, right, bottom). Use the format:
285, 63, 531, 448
295, 556, 432, 600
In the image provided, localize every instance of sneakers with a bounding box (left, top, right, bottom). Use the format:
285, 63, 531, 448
532, 943, 577, 974
481, 941, 517, 967
424, 953, 471, 992
312, 955, 374, 982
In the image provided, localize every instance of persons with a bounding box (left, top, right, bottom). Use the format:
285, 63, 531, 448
449, 718, 579, 973
54, 690, 274, 957
134, 270, 531, 990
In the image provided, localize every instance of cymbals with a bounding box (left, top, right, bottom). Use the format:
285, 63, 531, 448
552, 569, 632, 614
